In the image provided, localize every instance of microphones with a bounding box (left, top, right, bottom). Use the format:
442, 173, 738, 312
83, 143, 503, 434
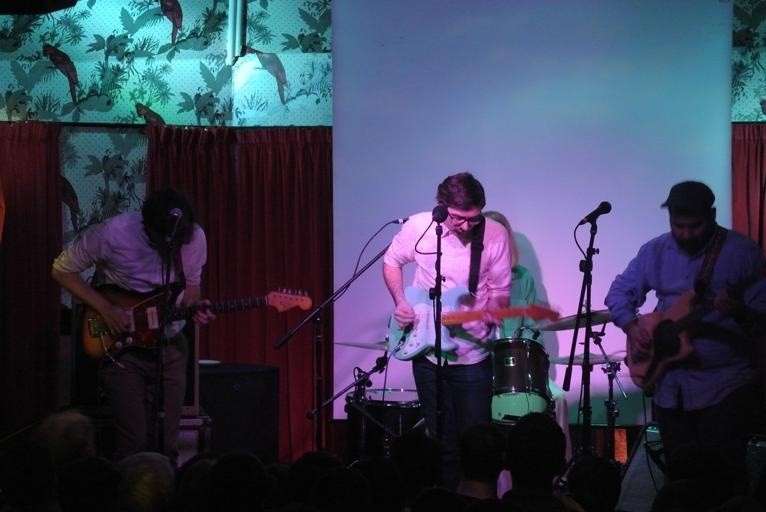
432, 206, 448, 227
167, 208, 182, 249
578, 201, 612, 226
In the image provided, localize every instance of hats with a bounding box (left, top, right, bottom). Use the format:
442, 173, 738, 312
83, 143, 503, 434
661, 181, 715, 211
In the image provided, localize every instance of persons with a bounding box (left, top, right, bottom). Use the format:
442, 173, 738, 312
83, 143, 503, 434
382, 172, 511, 487
48, 192, 215, 452
1, 413, 765, 512
486, 210, 571, 470
603, 181, 765, 465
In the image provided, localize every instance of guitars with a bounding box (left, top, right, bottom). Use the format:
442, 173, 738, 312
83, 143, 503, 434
626, 264, 766, 388
388, 287, 558, 360
82, 282, 313, 356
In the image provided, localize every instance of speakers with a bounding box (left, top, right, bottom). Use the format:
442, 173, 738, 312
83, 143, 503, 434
185, 363, 280, 461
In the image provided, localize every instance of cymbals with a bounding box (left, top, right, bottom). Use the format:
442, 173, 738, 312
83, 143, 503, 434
539, 309, 614, 331
330, 339, 386, 349
549, 354, 626, 365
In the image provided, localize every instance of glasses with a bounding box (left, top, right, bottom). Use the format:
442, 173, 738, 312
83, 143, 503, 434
448, 211, 483, 226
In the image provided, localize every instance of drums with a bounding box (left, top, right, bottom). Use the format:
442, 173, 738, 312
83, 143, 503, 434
492, 338, 555, 425
346, 388, 433, 475
